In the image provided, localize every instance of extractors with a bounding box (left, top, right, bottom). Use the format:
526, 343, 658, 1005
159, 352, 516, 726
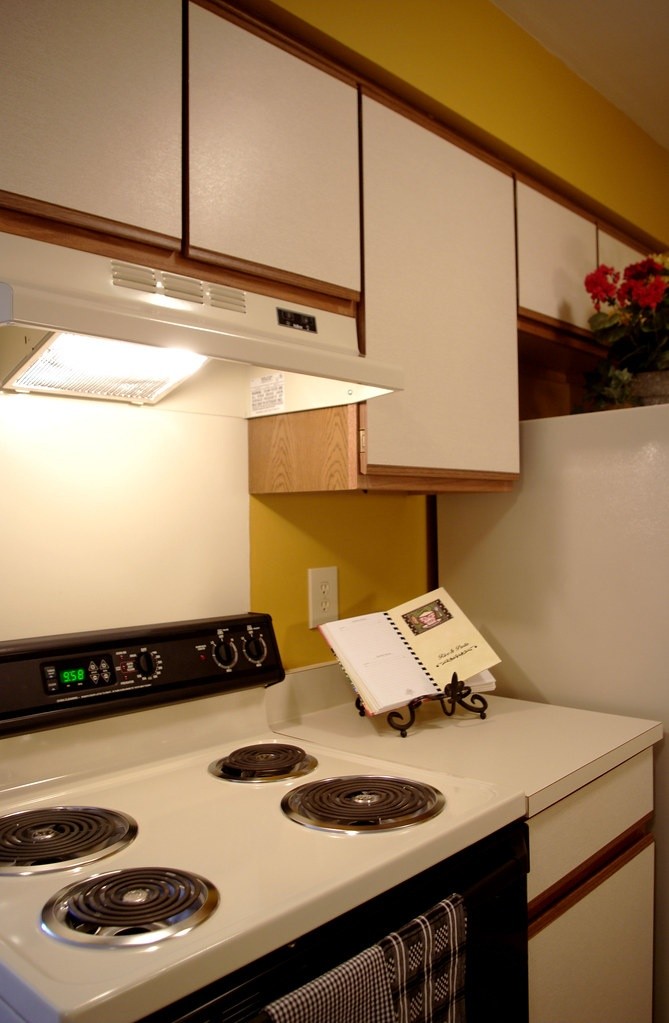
1, 227, 407, 418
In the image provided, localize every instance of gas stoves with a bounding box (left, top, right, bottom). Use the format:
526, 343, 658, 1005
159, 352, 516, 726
0, 729, 528, 1023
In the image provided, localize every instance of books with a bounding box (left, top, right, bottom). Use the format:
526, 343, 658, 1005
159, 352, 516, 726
317, 587, 502, 718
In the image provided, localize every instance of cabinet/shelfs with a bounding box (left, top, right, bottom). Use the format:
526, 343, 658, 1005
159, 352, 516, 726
0, 1, 657, 495
271, 695, 663, 1023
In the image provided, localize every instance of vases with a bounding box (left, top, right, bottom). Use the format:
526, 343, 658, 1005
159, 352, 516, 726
628, 373, 669, 404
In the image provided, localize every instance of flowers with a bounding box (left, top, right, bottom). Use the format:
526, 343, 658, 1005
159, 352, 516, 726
580, 254, 669, 408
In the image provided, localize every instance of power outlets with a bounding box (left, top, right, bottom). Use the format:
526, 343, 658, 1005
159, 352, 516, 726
308, 565, 338, 630
